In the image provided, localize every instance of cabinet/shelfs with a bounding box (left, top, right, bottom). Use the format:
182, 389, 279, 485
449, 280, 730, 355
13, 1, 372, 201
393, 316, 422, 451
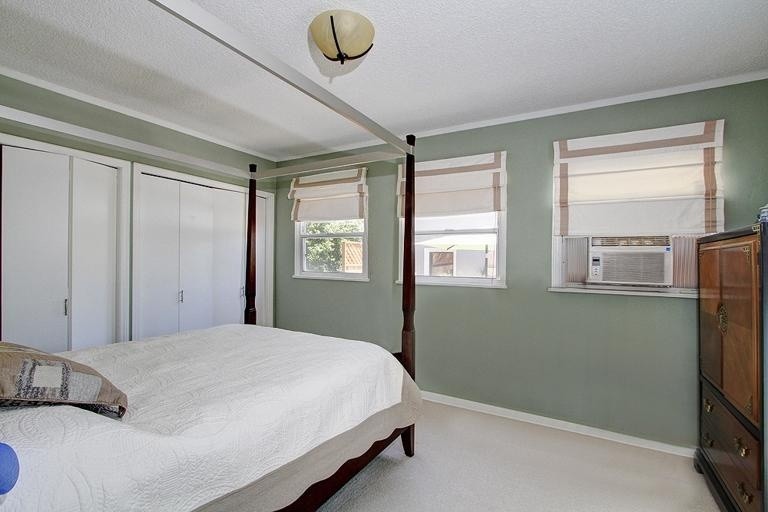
0, 132, 276, 356
692, 219, 768, 511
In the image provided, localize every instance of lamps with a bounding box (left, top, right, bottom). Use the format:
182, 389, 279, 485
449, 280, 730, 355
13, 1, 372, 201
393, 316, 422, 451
310, 8, 375, 66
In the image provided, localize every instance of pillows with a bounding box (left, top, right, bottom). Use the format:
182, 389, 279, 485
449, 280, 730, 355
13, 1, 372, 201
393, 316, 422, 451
0, 341, 128, 418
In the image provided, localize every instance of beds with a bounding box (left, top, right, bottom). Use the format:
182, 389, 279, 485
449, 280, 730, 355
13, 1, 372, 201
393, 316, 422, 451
0, 323, 410, 512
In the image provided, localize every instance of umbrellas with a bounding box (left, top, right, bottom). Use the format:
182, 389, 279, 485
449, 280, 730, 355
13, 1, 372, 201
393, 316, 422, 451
414, 234, 497, 277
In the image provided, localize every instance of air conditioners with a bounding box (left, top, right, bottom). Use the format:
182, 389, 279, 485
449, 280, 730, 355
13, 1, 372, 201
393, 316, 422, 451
585, 245, 675, 288
